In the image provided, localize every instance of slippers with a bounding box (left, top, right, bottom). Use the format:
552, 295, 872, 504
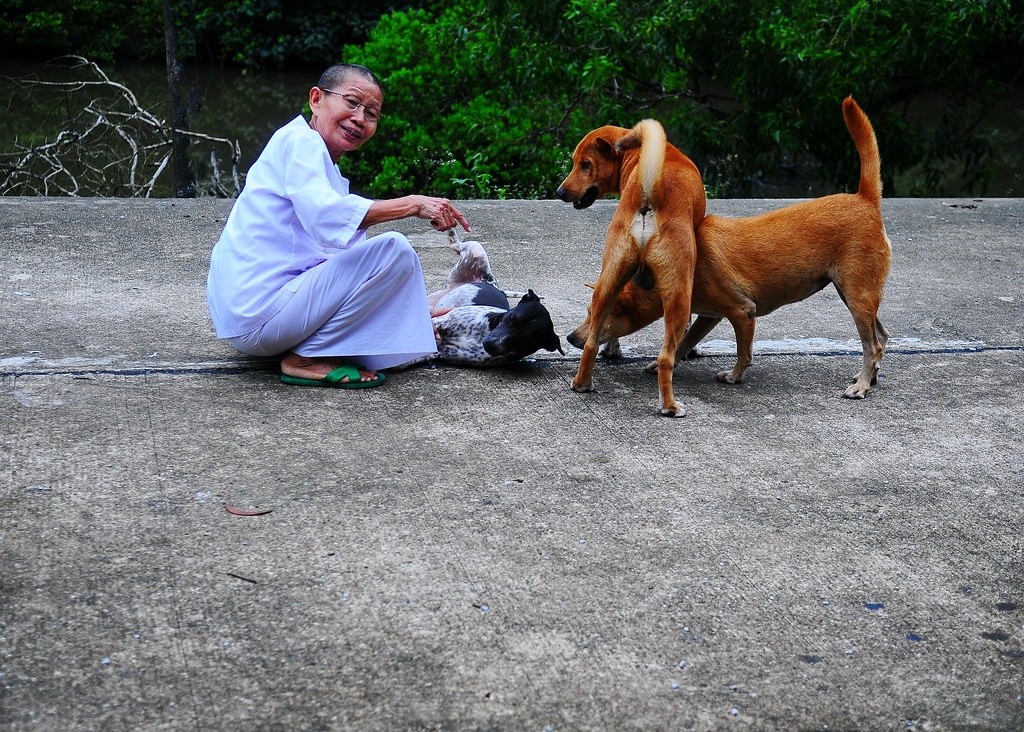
281, 361, 385, 389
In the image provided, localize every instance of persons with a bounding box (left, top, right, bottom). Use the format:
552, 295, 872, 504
205, 63, 474, 390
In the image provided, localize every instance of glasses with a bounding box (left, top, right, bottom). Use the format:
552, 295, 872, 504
321, 88, 384, 122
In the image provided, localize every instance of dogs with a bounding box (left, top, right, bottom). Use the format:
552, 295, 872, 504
389, 228, 566, 370
555, 95, 891, 417
